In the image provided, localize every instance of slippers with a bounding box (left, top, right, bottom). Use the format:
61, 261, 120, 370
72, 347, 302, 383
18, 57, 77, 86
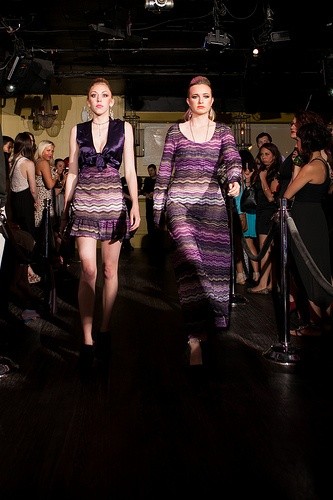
290, 326, 320, 338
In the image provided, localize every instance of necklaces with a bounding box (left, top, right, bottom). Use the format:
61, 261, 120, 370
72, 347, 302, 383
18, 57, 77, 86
190, 119, 209, 167
92, 120, 109, 125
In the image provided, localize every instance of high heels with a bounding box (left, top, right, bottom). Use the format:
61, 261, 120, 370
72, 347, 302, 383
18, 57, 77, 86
188, 347, 205, 376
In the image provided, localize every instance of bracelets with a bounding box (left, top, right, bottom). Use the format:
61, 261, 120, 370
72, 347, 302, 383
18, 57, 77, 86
263, 187, 269, 193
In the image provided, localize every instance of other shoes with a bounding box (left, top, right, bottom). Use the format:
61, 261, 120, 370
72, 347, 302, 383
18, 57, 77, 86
247, 287, 269, 294
79, 339, 97, 367
236, 282, 246, 285
96, 323, 111, 348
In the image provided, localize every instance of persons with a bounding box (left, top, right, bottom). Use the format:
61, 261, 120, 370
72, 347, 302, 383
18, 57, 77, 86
52, 158, 68, 267
33, 139, 59, 227
0, 123, 8, 270
142, 164, 165, 266
3, 136, 14, 192
59, 77, 141, 352
153, 77, 242, 380
275, 110, 333, 336
120, 169, 143, 252
8, 132, 37, 292
226, 132, 285, 294
